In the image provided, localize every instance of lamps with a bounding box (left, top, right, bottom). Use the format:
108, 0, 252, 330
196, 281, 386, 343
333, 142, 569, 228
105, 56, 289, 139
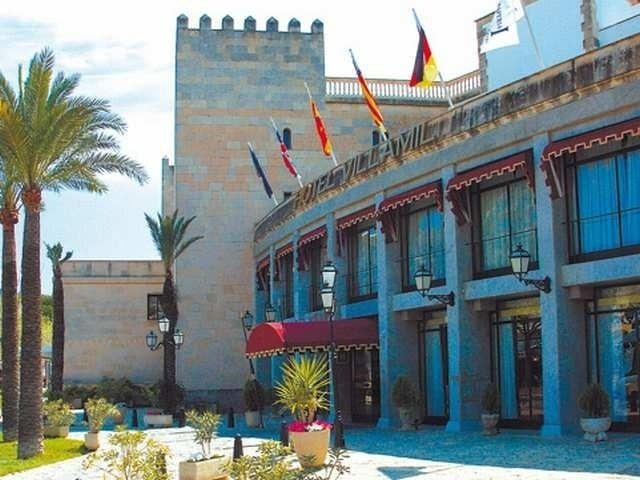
414, 241, 551, 308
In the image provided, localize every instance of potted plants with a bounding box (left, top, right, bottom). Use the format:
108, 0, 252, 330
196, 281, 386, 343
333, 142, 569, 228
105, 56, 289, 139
43, 347, 340, 480
389, 371, 614, 444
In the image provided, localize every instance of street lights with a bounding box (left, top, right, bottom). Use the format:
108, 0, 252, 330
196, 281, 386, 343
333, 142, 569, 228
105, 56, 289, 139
145, 316, 183, 389
320, 260, 347, 450
240, 310, 264, 427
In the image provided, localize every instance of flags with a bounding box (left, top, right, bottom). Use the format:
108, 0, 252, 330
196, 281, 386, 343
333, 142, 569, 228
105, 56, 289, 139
350, 52, 387, 135
270, 118, 299, 177
305, 86, 333, 160
409, 16, 438, 89
247, 143, 273, 199
